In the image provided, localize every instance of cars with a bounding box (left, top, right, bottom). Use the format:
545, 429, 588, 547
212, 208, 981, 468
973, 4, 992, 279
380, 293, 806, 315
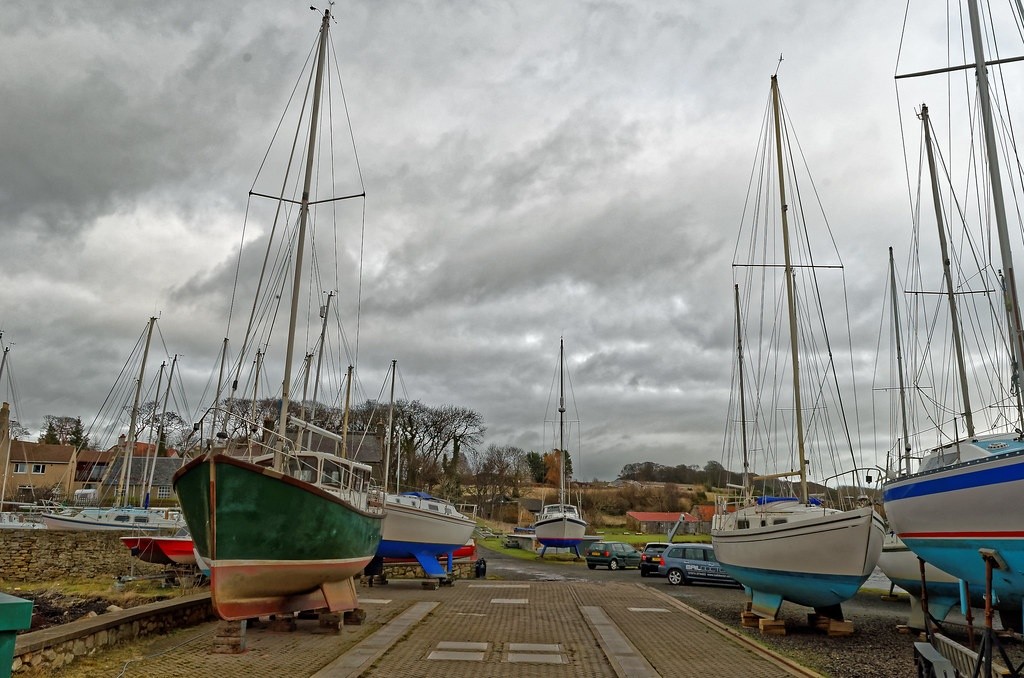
657, 543, 745, 590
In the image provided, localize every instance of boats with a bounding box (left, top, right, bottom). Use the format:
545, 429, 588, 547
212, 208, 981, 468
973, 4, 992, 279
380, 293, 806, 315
533, 335, 587, 548
364, 491, 477, 578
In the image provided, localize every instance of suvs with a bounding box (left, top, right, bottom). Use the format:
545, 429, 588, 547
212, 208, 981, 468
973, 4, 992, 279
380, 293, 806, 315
586, 541, 642, 570
639, 542, 674, 577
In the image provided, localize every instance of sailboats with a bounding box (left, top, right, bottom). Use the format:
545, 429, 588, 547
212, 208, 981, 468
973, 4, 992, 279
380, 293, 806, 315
871, 103, 1011, 604
881, 0, 1024, 604
0, 309, 196, 567
171, 0, 388, 622
711, 51, 887, 623
